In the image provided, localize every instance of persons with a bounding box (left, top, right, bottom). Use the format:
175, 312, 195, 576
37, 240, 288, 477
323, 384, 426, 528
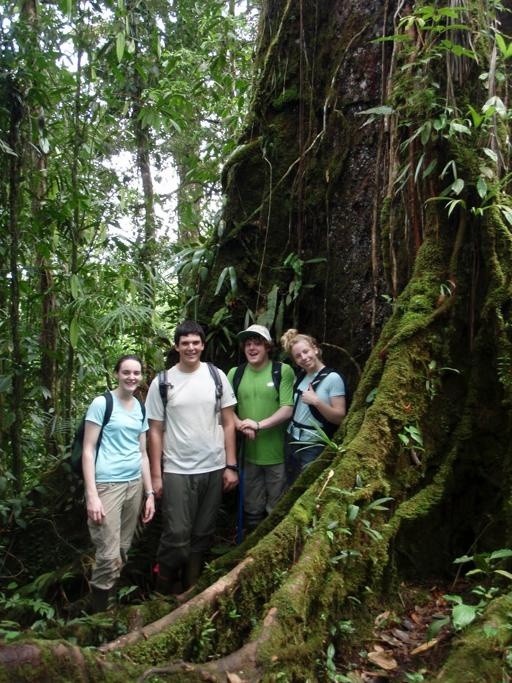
226, 324, 295, 533
280, 328, 346, 486
144, 319, 240, 596
81, 354, 155, 613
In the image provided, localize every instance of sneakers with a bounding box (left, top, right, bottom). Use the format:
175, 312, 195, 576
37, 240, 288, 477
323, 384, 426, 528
93, 584, 116, 611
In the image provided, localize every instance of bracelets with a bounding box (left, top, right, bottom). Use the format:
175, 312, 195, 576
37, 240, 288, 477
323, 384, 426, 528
145, 491, 155, 496
226, 464, 239, 471
257, 422, 259, 431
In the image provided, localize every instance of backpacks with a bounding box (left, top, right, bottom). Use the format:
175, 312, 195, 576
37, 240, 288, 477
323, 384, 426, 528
292, 367, 345, 438
71, 391, 146, 479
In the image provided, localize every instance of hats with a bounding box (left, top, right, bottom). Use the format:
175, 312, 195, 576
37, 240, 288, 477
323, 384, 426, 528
236, 324, 272, 345
